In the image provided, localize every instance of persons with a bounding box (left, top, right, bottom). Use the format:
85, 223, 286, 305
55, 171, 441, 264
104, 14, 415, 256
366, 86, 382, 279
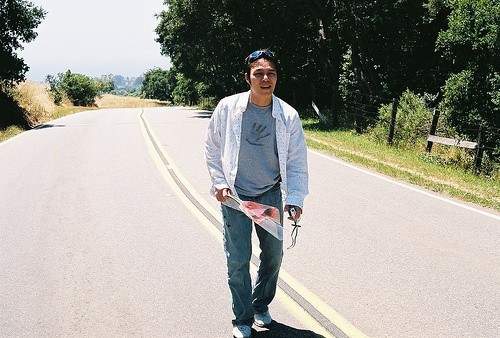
204, 50, 309, 338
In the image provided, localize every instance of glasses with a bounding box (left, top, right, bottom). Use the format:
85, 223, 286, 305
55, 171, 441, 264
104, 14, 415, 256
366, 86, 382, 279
247, 49, 276, 63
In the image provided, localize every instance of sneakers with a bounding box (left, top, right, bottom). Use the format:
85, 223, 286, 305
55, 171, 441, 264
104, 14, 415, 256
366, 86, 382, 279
232, 324, 251, 338
253, 310, 272, 327
